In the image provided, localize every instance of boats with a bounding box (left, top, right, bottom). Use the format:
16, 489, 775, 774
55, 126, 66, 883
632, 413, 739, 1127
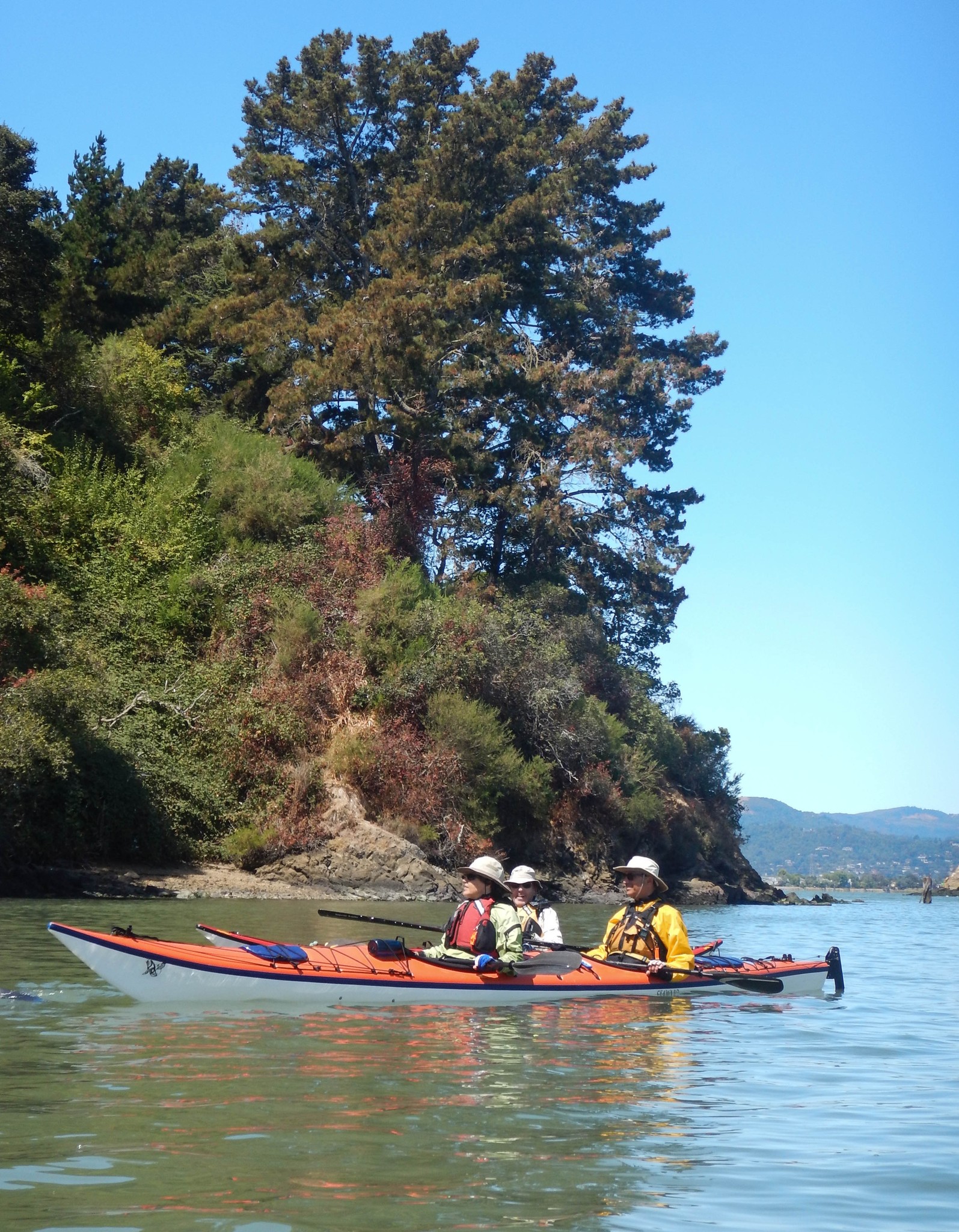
194, 923, 724, 973
46, 919, 845, 1008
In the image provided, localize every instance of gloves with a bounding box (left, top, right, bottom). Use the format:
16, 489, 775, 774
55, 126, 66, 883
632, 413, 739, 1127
473, 954, 503, 973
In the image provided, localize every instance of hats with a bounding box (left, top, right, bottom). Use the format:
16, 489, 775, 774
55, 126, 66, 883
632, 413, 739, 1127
457, 856, 513, 894
613, 855, 668, 893
502, 865, 543, 892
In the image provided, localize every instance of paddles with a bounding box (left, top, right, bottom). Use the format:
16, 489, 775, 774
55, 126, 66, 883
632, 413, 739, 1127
317, 908, 596, 954
600, 960, 786, 995
442, 950, 582, 975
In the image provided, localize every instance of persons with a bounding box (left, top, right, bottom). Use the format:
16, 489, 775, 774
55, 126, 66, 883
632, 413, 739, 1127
580, 856, 695, 983
411, 856, 523, 977
503, 865, 563, 960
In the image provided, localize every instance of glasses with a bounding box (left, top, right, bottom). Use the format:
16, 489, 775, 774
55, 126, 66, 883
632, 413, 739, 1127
462, 873, 479, 881
509, 882, 533, 889
621, 872, 647, 881
